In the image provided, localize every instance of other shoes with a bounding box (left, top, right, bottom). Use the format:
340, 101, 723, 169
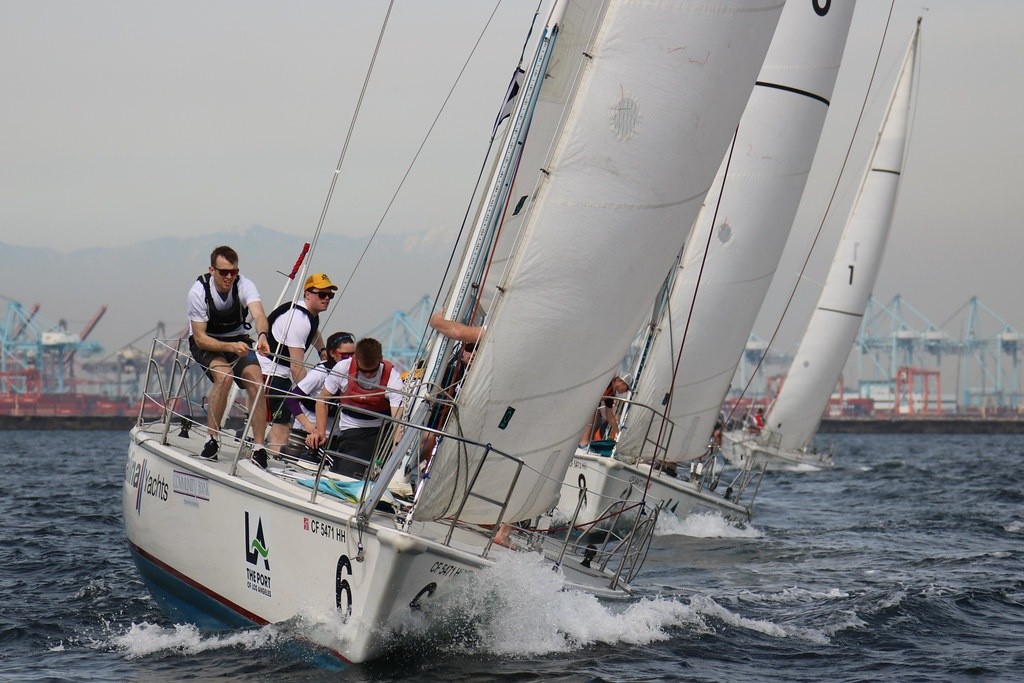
272, 454, 289, 465
239, 436, 255, 447
576, 445, 601, 456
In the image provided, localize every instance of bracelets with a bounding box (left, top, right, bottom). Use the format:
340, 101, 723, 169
258, 332, 268, 339
318, 348, 326, 356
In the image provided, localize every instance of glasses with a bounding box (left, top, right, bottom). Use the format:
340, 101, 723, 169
211, 265, 239, 276
356, 360, 380, 373
308, 290, 334, 299
332, 349, 355, 360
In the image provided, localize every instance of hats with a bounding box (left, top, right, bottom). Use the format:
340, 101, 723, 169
619, 371, 634, 393
304, 273, 338, 291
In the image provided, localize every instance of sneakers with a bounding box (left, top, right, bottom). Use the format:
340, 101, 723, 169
199, 435, 219, 460
251, 448, 270, 470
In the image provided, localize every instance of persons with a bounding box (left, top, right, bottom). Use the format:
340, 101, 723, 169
188, 246, 271, 471
755, 409, 764, 428
714, 428, 722, 445
409, 307, 519, 551
256, 273, 338, 453
578, 372, 633, 456
285, 332, 407, 479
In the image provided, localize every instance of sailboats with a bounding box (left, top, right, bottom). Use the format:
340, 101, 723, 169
551, 0, 856, 538
719, 16, 923, 474
121, 0, 787, 671
